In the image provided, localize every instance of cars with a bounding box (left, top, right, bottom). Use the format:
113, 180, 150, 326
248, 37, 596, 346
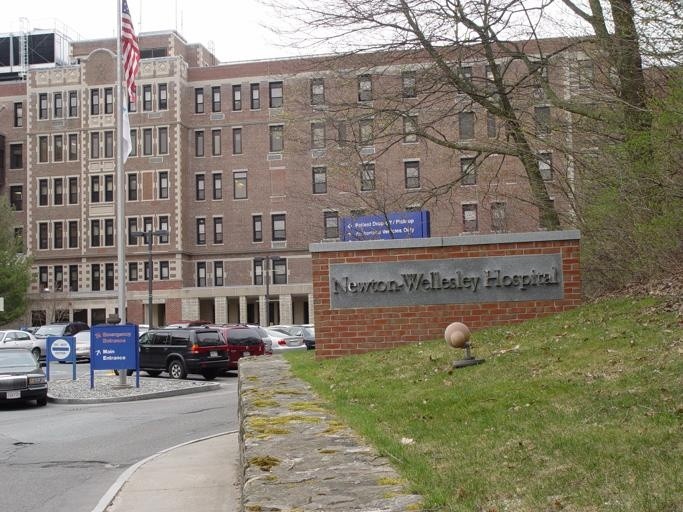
0, 322, 90, 405
114, 320, 315, 380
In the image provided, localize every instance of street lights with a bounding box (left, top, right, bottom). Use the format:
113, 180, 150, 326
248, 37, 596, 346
131, 230, 168, 330
255, 255, 280, 325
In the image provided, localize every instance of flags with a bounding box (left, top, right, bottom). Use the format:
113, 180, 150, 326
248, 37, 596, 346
118, 94, 132, 163
118, 0, 143, 100
